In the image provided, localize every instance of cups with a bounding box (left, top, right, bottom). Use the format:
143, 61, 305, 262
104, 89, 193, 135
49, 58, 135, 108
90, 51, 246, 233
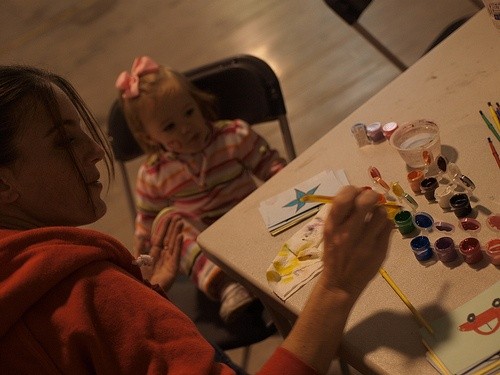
390, 119, 442, 169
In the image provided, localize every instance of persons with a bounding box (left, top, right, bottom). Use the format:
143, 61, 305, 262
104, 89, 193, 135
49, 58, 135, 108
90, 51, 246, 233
114, 53, 291, 328
0, 65, 394, 375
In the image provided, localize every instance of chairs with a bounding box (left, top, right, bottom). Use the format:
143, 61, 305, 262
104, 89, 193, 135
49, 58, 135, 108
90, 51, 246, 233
108, 54, 354, 375
322, 0, 486, 73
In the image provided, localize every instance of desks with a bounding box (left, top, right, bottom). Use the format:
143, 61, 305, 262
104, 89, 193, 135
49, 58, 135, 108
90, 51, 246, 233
197, 7, 500, 375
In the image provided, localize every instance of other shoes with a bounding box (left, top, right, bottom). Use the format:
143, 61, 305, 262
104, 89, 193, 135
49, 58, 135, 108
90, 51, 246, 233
220, 280, 258, 328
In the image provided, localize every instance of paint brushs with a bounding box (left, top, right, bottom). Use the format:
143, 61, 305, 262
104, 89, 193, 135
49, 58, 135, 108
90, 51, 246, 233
478, 108, 500, 142
379, 266, 434, 335
423, 339, 452, 375
487, 136, 499, 168
487, 102, 500, 132
299, 194, 406, 209
